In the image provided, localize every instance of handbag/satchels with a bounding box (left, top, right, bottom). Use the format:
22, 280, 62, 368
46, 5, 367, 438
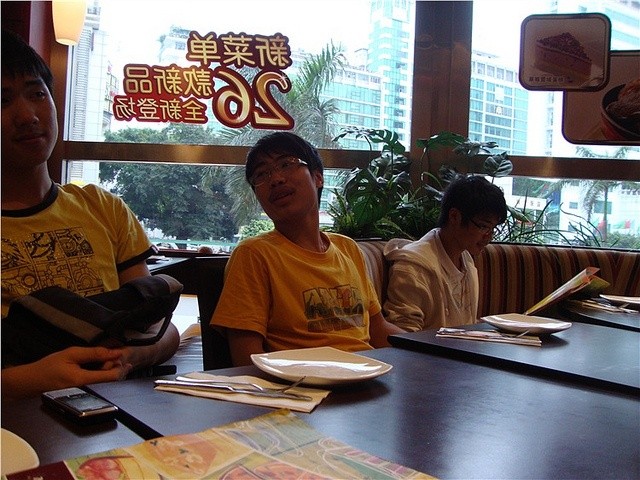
1, 274, 184, 368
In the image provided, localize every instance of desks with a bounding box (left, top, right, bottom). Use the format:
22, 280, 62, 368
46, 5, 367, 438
559, 299, 640, 331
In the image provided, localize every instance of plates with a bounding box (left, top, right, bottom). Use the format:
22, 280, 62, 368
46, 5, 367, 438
480, 313, 572, 335
250, 346, 394, 385
599, 294, 640, 311
1, 428, 40, 474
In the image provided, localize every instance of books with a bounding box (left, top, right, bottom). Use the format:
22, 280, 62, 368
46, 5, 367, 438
524, 268, 609, 321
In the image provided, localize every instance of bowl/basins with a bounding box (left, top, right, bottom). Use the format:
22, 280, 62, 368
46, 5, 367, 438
600, 82, 640, 141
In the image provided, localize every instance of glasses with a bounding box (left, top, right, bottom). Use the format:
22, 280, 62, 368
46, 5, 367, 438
467, 216, 498, 236
249, 156, 308, 187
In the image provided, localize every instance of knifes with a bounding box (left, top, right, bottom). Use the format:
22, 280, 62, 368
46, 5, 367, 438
439, 333, 542, 344
153, 379, 313, 402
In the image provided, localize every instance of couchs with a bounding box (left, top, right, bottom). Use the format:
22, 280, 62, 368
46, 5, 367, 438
351, 240, 640, 325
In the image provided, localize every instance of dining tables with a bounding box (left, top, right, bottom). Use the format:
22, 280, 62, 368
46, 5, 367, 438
1, 322, 640, 480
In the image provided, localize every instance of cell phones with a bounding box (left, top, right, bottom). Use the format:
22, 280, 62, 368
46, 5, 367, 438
41, 387, 118, 421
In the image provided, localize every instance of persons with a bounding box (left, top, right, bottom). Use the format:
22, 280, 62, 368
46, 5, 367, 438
382, 175, 508, 333
209, 132, 410, 368
1, 32, 181, 396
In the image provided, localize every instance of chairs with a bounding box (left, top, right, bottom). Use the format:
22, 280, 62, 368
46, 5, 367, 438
184, 258, 264, 370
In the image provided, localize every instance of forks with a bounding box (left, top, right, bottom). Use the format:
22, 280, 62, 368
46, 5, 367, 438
436, 329, 530, 338
175, 375, 307, 394
